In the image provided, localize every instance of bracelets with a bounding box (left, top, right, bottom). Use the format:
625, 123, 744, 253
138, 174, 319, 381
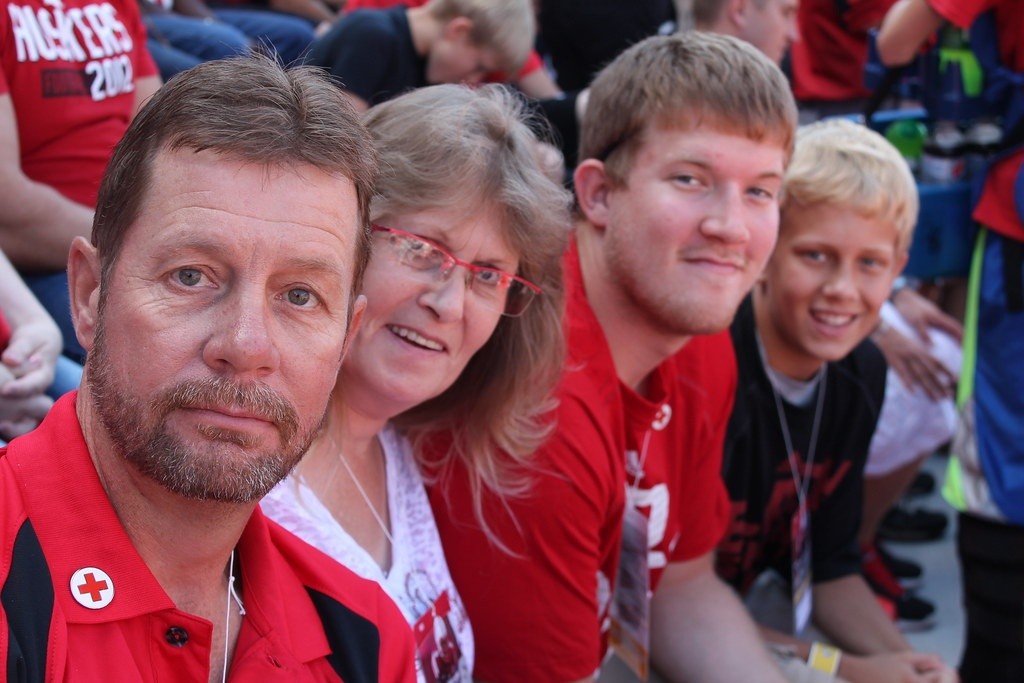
805, 638, 844, 676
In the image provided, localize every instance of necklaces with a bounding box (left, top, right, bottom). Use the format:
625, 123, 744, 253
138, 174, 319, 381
327, 434, 395, 546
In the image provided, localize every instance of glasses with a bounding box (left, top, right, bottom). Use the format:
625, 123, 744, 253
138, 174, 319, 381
368, 224, 543, 319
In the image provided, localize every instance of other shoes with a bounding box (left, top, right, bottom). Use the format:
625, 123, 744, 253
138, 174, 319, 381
904, 470, 938, 500
881, 506, 950, 545
890, 596, 937, 629
881, 551, 926, 587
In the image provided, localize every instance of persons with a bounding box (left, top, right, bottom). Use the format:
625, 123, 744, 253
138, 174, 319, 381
259, 77, 568, 676
1, 50, 418, 683
0, 0, 1024, 624
715, 116, 962, 683
391, 26, 787, 683
